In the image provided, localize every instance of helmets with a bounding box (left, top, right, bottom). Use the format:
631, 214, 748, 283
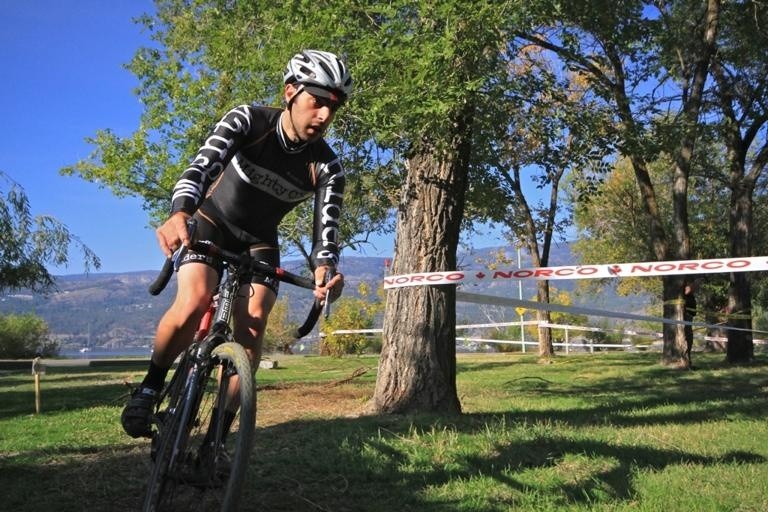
284, 48, 354, 99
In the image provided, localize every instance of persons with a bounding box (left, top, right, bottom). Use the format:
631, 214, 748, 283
684, 289, 698, 364
119, 47, 353, 479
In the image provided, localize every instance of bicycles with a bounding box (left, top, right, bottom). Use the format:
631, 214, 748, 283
109, 218, 339, 512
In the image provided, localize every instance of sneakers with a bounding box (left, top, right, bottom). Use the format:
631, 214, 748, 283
120, 382, 158, 438
197, 445, 217, 481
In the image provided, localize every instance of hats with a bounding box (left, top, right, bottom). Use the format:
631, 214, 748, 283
295, 82, 345, 106
